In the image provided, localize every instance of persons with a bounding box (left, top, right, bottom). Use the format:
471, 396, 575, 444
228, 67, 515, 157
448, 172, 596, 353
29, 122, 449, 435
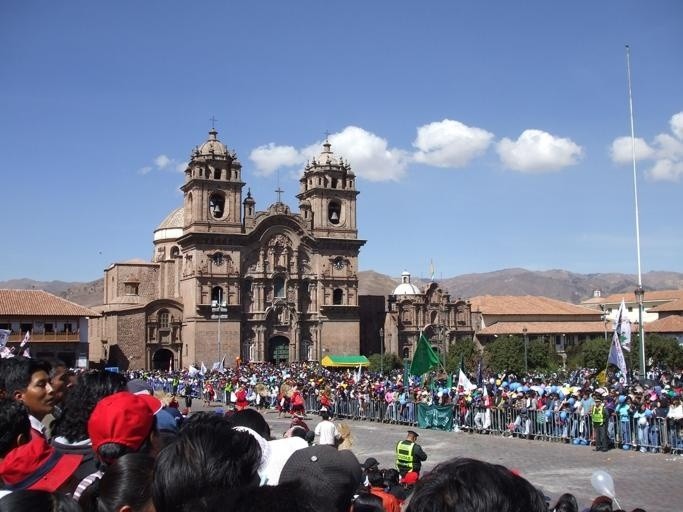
1, 327, 683, 511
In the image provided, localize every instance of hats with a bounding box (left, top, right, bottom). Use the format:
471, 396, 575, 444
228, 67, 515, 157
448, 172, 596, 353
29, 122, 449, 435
278, 444, 362, 512
0, 427, 84, 493
365, 458, 379, 469
228, 409, 308, 486
87, 378, 184, 465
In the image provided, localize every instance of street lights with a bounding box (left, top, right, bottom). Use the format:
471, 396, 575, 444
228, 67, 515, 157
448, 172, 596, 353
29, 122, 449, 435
562, 332, 566, 352
378, 327, 384, 379
521, 325, 528, 373
632, 286, 647, 386
210, 290, 228, 362
624, 44, 646, 380
599, 303, 610, 341
